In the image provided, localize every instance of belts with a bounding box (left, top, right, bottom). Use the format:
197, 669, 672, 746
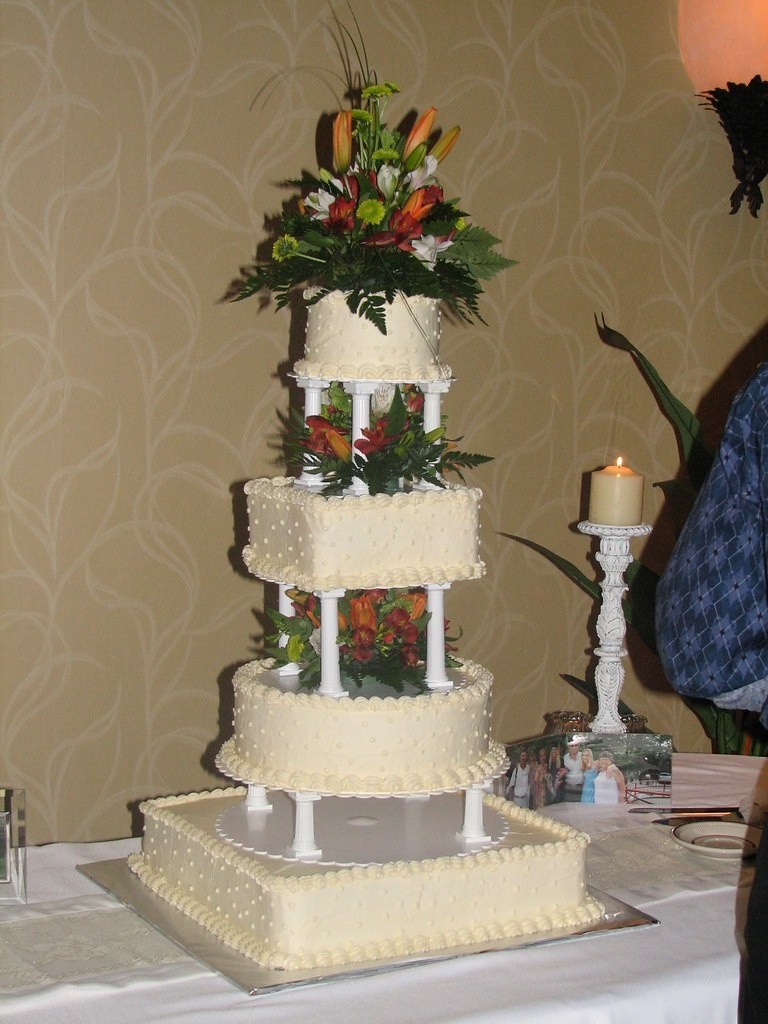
514, 792, 527, 799
565, 783, 583, 788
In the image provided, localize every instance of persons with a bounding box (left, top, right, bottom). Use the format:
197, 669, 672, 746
658, 360, 768, 1024
508, 736, 626, 810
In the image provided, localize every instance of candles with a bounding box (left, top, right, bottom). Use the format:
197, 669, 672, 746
587, 456, 645, 526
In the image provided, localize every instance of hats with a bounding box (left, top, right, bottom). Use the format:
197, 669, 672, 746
566, 735, 581, 746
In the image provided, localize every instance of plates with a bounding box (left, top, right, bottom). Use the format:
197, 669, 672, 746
671, 822, 763, 859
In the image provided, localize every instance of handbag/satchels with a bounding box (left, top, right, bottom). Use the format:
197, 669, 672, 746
507, 766, 518, 801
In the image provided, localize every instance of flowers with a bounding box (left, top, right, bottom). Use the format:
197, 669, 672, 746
274, 380, 496, 496
261, 587, 464, 695
230, 0, 522, 339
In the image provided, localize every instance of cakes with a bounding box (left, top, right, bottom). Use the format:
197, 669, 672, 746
127, 785, 605, 971
240, 475, 487, 594
293, 286, 452, 381
216, 658, 507, 794
